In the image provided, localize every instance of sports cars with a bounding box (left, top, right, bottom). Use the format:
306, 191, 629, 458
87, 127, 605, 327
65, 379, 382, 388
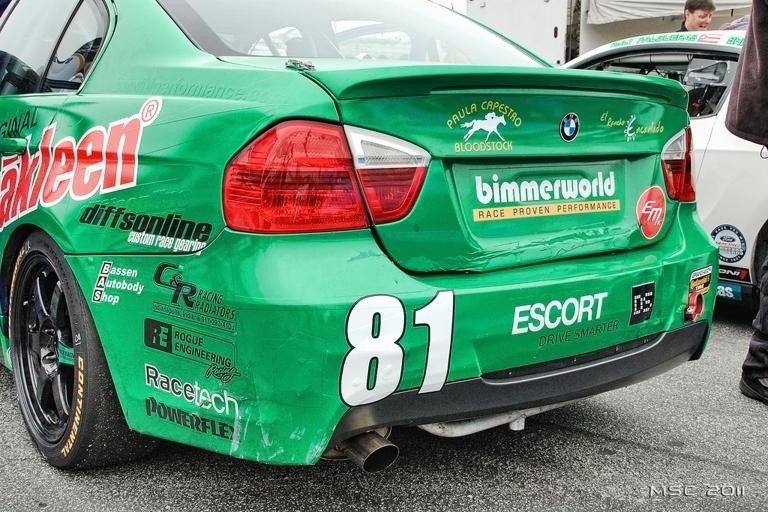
0, 0, 720, 472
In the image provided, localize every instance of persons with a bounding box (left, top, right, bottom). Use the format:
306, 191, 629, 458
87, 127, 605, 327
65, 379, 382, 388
725, 0, 768, 407
673, 1, 717, 30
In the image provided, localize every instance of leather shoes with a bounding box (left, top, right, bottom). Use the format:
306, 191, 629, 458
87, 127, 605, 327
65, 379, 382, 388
739, 371, 768, 406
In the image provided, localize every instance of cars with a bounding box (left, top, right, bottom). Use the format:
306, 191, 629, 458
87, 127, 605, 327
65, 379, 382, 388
555, 29, 768, 304
